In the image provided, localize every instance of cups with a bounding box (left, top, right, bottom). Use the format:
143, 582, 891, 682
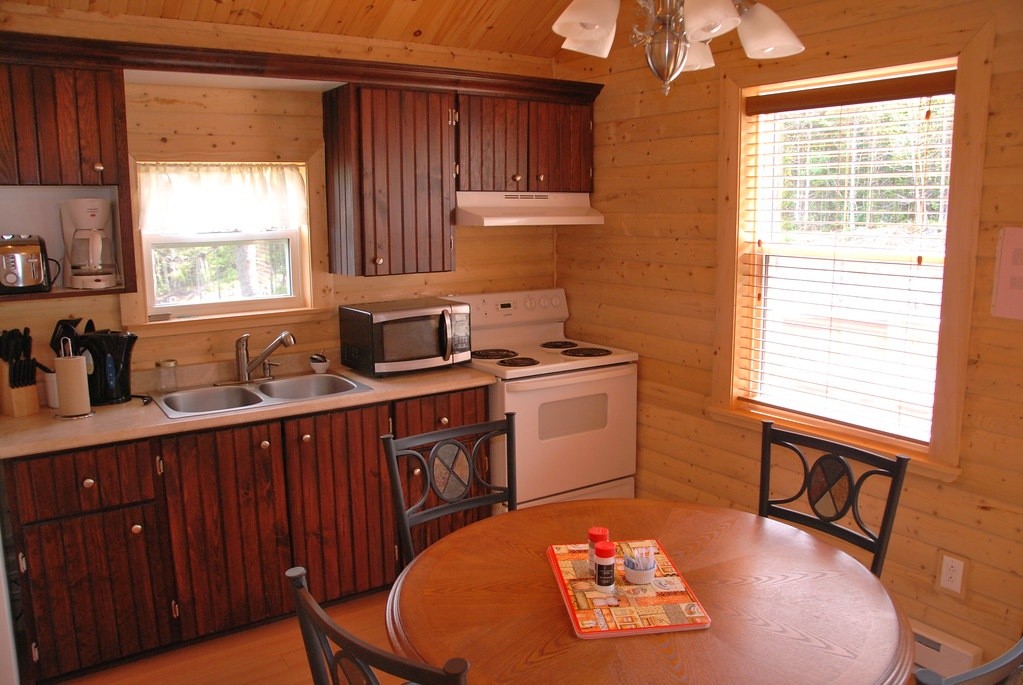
44, 372, 58, 409
623, 560, 657, 585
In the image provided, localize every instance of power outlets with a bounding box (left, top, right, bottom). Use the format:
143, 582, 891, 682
934, 548, 970, 601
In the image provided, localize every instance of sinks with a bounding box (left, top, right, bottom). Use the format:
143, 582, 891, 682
147, 384, 263, 418
259, 374, 374, 402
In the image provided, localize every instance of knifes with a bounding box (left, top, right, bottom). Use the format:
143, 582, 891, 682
0, 327, 37, 389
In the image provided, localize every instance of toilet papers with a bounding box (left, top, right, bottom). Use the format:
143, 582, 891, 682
53, 356, 90, 416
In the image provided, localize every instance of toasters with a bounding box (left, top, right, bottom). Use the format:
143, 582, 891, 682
0, 232, 52, 295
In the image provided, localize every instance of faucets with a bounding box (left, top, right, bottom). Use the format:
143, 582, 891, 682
212, 330, 296, 387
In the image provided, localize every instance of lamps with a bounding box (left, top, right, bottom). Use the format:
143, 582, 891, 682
550, 0, 805, 96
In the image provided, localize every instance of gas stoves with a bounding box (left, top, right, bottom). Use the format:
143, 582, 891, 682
438, 288, 639, 380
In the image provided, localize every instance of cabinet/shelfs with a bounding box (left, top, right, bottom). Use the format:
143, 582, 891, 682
0, 436, 177, 685
322, 58, 456, 278
389, 387, 491, 573
0, 29, 138, 303
456, 68, 605, 193
153, 402, 400, 648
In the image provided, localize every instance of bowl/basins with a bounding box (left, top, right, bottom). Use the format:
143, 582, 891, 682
310, 360, 331, 374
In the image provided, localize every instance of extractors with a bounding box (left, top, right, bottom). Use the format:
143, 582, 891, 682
455, 191, 605, 227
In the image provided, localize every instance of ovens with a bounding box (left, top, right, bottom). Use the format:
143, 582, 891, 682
486, 361, 637, 516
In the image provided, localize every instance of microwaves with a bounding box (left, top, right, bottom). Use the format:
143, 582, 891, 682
339, 295, 472, 377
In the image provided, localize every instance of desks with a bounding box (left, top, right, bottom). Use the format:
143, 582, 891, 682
384, 497, 916, 685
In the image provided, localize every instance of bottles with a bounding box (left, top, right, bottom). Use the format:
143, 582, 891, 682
593, 542, 616, 592
588, 527, 609, 576
156, 359, 177, 394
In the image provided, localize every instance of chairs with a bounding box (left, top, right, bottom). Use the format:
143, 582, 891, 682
758, 417, 911, 579
284, 566, 471, 685
380, 411, 518, 566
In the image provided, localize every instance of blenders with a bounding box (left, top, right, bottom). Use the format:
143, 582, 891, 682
56, 197, 118, 288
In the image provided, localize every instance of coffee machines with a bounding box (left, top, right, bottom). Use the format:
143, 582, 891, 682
79, 328, 137, 406
